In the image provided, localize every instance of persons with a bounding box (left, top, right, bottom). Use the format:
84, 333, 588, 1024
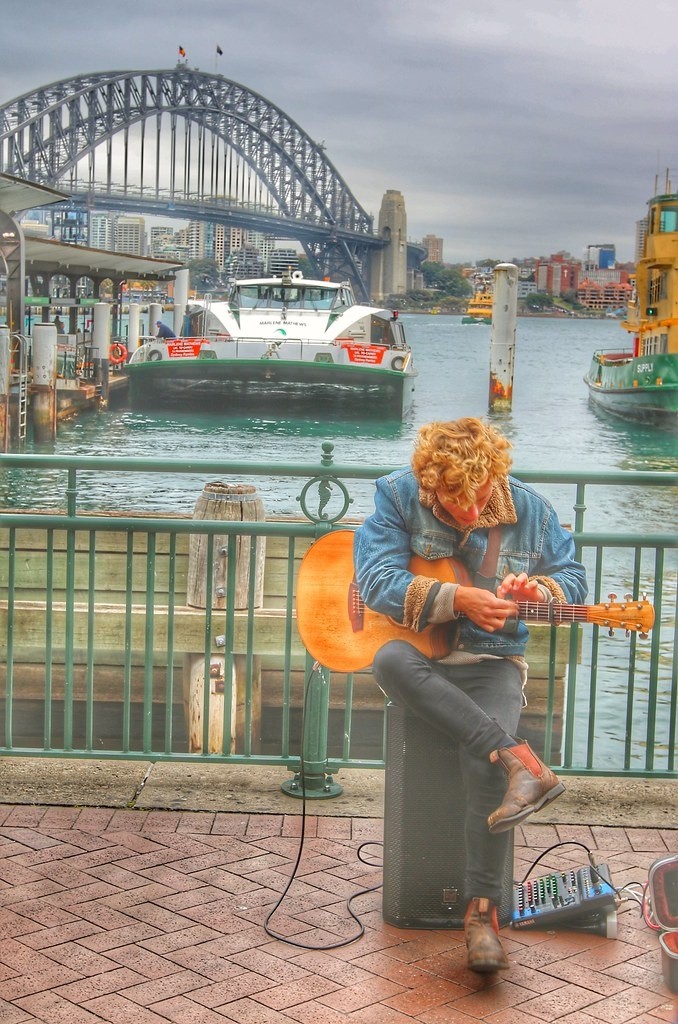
352, 417, 589, 971
52, 315, 65, 336
155, 320, 176, 339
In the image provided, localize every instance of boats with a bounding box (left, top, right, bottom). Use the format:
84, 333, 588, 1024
461, 284, 497, 326
583, 166, 678, 427
123, 264, 421, 419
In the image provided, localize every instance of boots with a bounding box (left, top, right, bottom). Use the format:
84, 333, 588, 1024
487, 736, 567, 833
464, 897, 510, 972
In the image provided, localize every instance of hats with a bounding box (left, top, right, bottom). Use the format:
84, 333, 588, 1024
155, 320, 162, 324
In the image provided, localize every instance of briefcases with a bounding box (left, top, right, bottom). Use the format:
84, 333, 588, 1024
643, 855, 678, 991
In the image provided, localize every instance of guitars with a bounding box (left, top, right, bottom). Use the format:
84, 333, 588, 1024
295, 529, 655, 675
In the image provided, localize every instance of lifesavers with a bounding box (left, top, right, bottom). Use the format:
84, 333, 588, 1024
392, 356, 405, 371
107, 343, 129, 364
146, 349, 162, 362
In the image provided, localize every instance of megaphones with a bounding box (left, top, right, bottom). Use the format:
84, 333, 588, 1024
293, 270, 303, 280
